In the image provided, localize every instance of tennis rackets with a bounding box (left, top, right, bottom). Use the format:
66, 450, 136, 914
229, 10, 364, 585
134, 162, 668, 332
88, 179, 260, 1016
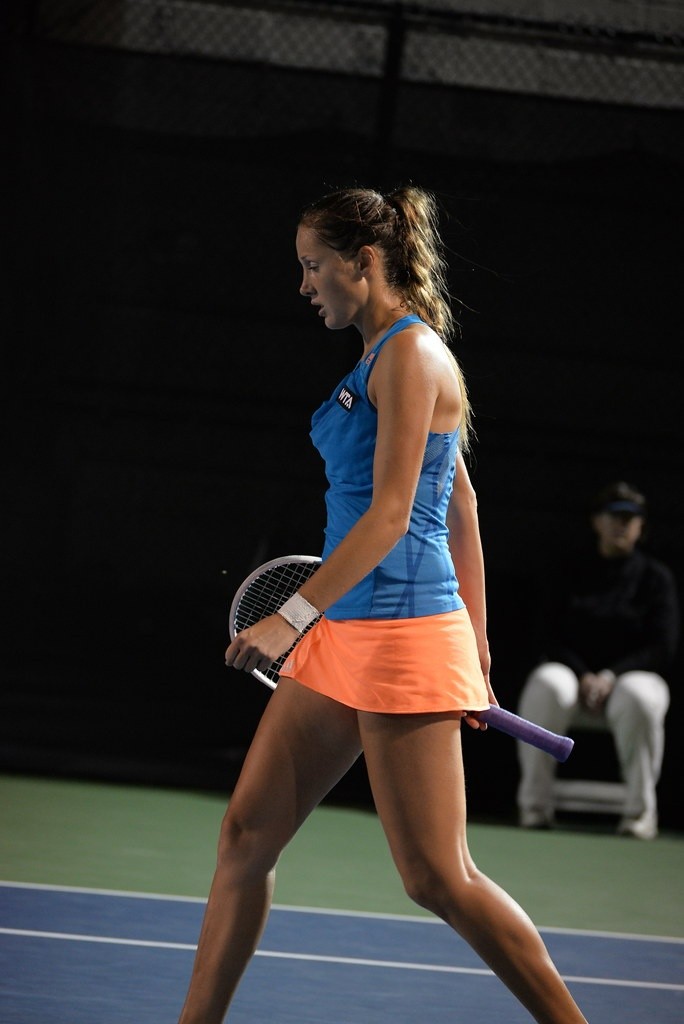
229, 553, 576, 764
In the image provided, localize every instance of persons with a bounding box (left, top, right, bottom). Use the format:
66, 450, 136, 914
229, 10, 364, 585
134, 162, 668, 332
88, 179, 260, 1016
518, 481, 684, 841
177, 185, 592, 1024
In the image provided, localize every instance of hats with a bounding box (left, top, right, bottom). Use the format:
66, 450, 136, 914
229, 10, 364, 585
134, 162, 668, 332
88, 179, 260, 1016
592, 482, 647, 520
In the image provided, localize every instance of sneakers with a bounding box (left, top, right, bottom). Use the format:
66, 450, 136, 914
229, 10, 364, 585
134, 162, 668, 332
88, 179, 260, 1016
617, 814, 658, 841
519, 786, 557, 829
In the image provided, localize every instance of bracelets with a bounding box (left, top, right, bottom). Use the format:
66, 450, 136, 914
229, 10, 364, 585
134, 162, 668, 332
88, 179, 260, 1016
277, 591, 322, 633
